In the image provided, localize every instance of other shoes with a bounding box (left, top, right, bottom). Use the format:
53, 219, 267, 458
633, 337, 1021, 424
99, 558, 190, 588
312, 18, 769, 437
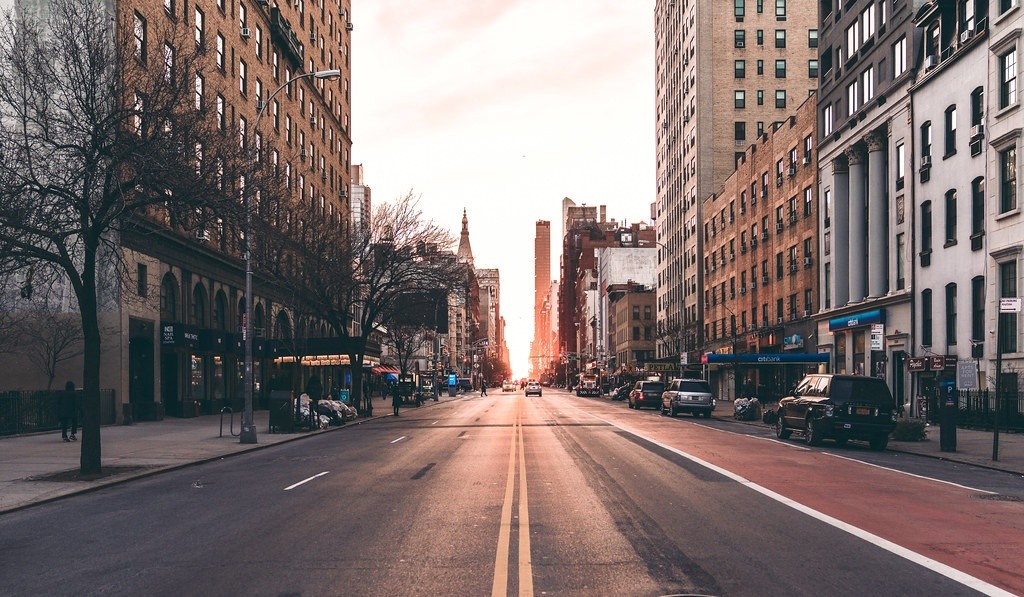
63, 436, 70, 442
70, 435, 78, 440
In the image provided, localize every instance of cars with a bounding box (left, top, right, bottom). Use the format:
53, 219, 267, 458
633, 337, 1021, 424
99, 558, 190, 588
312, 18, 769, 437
502, 379, 517, 392
525, 381, 543, 396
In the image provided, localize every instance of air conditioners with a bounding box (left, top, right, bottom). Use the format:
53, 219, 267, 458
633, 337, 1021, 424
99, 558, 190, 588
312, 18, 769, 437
195, 230, 211, 242
737, 42, 745, 46
759, 321, 767, 327
801, 310, 809, 318
259, 0, 270, 7
310, 33, 316, 41
704, 157, 812, 309
256, 99, 348, 197
748, 324, 757, 330
777, 316, 784, 324
241, 28, 252, 38
294, 0, 299, 7
662, 48, 692, 212
961, 29, 972, 43
921, 156, 931, 166
971, 125, 983, 137
925, 55, 937, 69
339, 8, 353, 53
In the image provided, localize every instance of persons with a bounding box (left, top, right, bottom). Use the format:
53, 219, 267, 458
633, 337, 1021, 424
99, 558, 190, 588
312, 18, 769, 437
520, 381, 524, 390
392, 386, 401, 415
382, 385, 387, 399
481, 383, 488, 397
569, 383, 573, 392
59, 381, 78, 442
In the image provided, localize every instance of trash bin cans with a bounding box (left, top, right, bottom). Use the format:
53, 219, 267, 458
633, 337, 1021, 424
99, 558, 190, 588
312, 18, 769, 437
603, 384, 609, 396
269, 390, 295, 434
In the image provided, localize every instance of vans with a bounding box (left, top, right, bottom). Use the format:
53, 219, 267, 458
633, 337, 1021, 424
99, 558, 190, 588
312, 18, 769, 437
442, 378, 473, 394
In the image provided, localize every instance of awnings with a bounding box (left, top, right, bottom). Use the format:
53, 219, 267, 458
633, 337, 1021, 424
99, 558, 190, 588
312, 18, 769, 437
372, 365, 401, 375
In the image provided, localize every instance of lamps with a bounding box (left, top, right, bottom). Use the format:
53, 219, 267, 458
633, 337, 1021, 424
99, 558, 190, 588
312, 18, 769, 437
901, 352, 909, 360
880, 355, 887, 364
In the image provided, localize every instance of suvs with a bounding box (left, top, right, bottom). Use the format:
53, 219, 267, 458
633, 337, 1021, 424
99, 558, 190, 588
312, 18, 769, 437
660, 379, 716, 419
776, 373, 897, 451
627, 380, 666, 411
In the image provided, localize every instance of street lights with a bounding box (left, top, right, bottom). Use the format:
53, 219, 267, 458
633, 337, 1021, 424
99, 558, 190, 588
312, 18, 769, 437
637, 238, 685, 378
239, 69, 342, 443
